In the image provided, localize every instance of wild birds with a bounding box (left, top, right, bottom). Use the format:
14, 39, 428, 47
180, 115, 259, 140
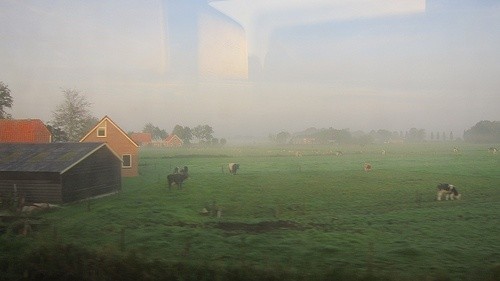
11, 192, 67, 218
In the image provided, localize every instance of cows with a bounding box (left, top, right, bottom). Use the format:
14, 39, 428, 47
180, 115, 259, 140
436, 183, 462, 201
228, 163, 240, 175
487, 147, 497, 156
364, 162, 372, 171
166, 166, 190, 190
